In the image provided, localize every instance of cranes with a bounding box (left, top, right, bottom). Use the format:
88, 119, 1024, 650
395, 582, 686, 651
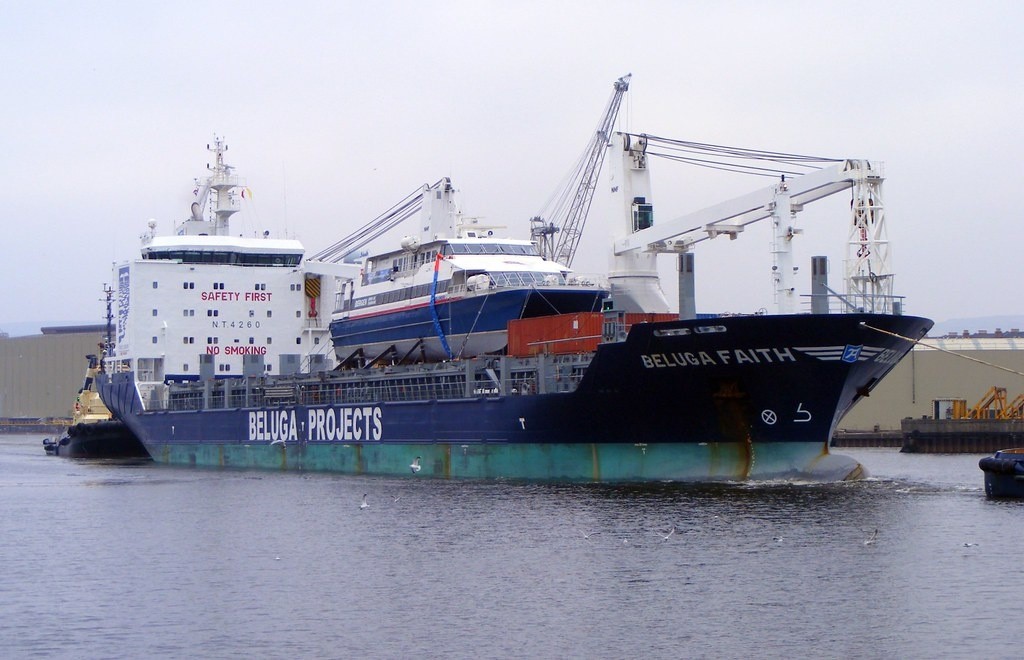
529, 73, 633, 269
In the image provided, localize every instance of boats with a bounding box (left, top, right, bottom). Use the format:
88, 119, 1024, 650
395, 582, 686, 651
42, 283, 146, 460
978, 446, 1024, 497
92, 71, 936, 484
